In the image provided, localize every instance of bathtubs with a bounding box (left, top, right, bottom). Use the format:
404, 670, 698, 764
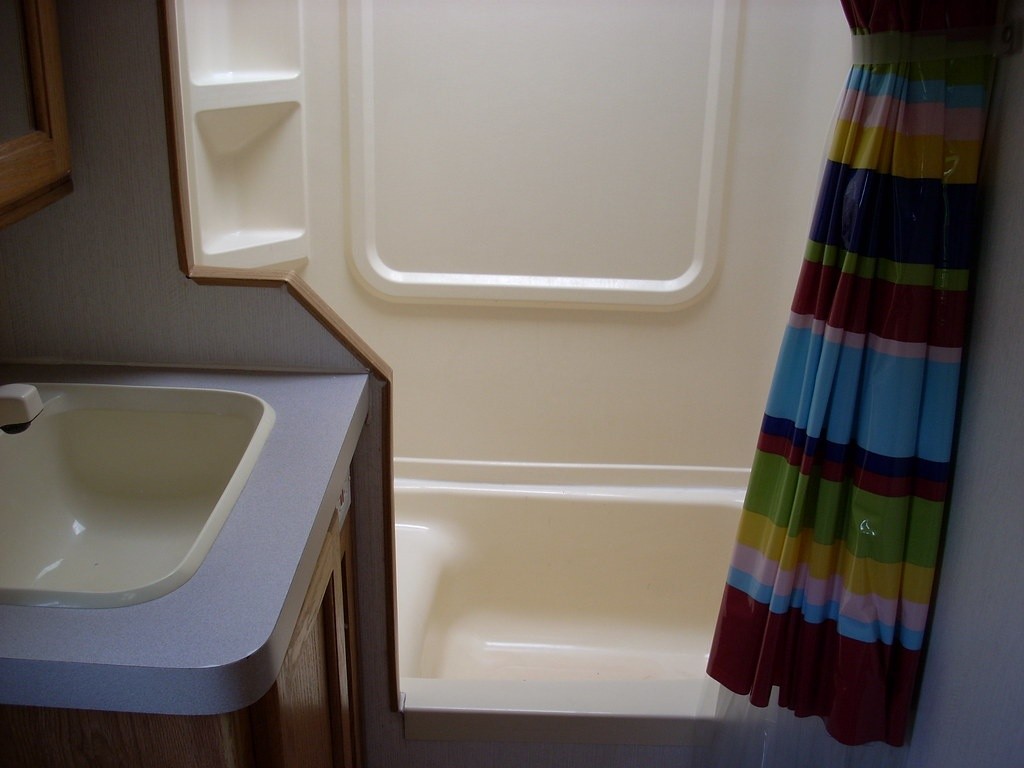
392, 479, 747, 717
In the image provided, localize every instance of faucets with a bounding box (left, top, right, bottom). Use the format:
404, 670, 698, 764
0, 383, 46, 435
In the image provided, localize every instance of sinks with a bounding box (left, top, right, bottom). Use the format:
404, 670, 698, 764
0, 382, 278, 611
